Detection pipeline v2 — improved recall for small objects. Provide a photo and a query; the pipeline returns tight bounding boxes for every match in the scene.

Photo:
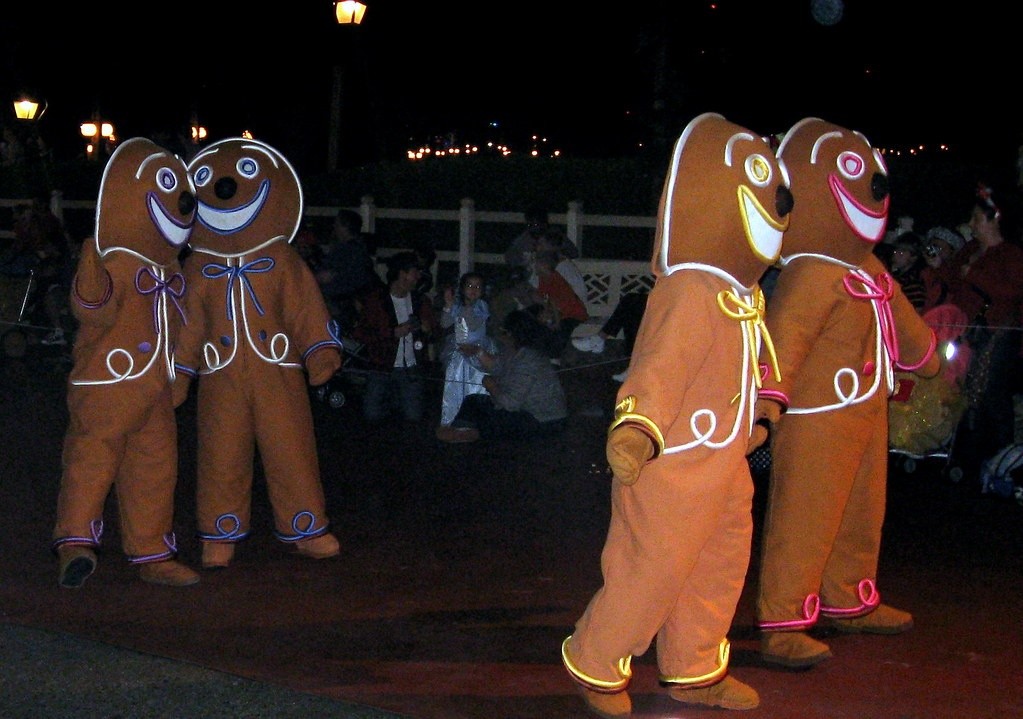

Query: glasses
[464,283,482,290]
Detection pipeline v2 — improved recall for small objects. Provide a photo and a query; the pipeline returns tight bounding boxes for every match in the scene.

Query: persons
[0,121,120,380]
[288,205,652,462]
[867,181,1023,427]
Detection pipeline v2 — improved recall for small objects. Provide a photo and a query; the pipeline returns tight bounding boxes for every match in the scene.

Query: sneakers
[571,336,604,354]
[612,369,629,382]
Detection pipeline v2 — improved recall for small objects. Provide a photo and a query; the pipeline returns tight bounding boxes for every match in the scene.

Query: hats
[888,232,920,252]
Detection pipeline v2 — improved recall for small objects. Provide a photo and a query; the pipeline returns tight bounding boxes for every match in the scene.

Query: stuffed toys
[53,137,343,588]
[562,113,939,719]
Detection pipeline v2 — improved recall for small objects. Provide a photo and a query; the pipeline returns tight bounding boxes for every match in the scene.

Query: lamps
[333,0,367,25]
[12,97,48,120]
